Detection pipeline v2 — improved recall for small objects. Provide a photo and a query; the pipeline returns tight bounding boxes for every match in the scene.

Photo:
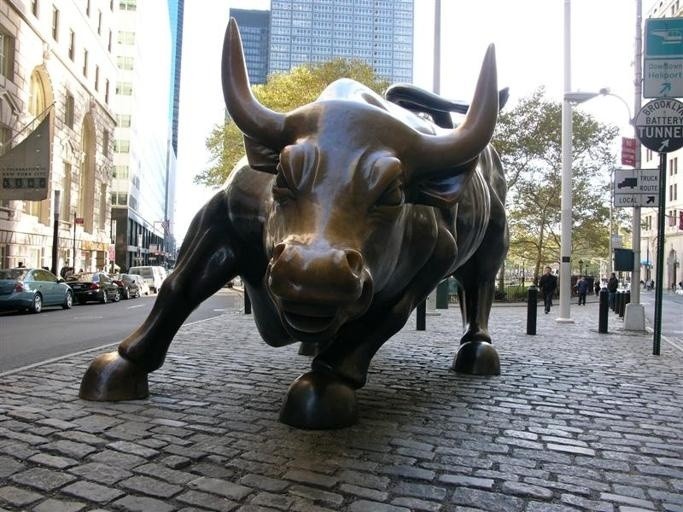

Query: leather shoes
[544,310,551,314]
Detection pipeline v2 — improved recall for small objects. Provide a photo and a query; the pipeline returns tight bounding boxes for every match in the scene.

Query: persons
[573,275,601,306]
[538,266,558,314]
[60,261,74,280]
[607,273,618,311]
[16,261,26,268]
[619,276,654,291]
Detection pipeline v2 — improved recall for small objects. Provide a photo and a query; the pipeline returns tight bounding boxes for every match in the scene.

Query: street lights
[563,87,642,303]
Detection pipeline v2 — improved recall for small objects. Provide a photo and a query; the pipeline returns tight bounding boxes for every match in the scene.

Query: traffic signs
[635,16,682,152]
[614,169,660,207]
[621,136,635,168]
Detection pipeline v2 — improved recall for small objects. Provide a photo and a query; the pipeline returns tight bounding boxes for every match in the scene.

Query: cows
[78,15,512,432]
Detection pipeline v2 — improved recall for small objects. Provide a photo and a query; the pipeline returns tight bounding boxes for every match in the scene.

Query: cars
[1,266,168,313]
